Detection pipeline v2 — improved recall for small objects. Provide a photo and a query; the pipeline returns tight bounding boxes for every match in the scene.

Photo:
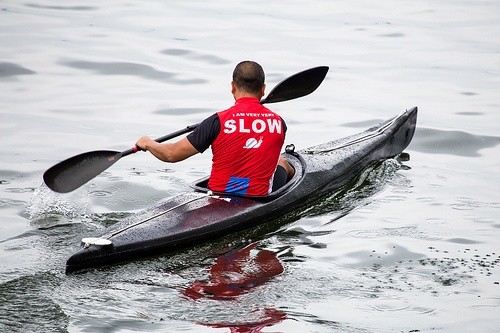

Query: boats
[64,106,418,273]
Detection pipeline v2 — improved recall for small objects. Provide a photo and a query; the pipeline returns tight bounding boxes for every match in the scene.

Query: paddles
[42,65,330,194]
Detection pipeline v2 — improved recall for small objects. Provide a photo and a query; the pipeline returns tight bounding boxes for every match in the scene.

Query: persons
[135,61,295,197]
